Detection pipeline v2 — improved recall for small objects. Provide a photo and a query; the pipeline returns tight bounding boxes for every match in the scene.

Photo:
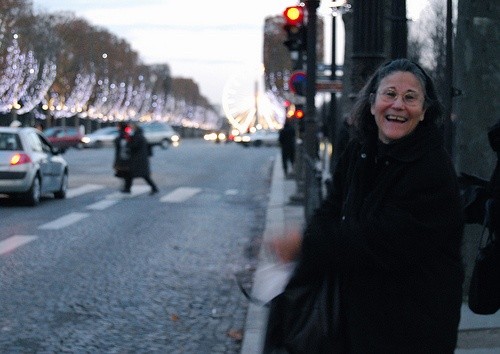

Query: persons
[278,121,296,174]
[262,57,467,354]
[112,121,160,196]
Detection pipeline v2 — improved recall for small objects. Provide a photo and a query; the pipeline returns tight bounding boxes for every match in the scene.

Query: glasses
[373,86,425,105]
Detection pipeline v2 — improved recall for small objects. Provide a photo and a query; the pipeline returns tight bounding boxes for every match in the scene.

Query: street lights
[326,0,342,140]
[7,98,21,121]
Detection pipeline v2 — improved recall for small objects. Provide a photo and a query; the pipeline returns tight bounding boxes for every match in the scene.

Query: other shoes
[118,186,130,192]
[149,185,158,195]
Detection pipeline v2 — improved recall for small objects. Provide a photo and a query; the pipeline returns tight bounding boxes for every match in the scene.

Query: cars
[139,123,180,148]
[238,131,280,146]
[0,127,69,206]
[83,126,120,148]
[42,127,84,150]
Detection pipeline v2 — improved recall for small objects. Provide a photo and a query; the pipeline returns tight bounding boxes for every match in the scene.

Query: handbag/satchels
[468,209,500,314]
[266,276,352,354]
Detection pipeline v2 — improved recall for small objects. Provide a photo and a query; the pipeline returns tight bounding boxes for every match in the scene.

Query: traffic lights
[294,105,304,138]
[283,7,307,51]
